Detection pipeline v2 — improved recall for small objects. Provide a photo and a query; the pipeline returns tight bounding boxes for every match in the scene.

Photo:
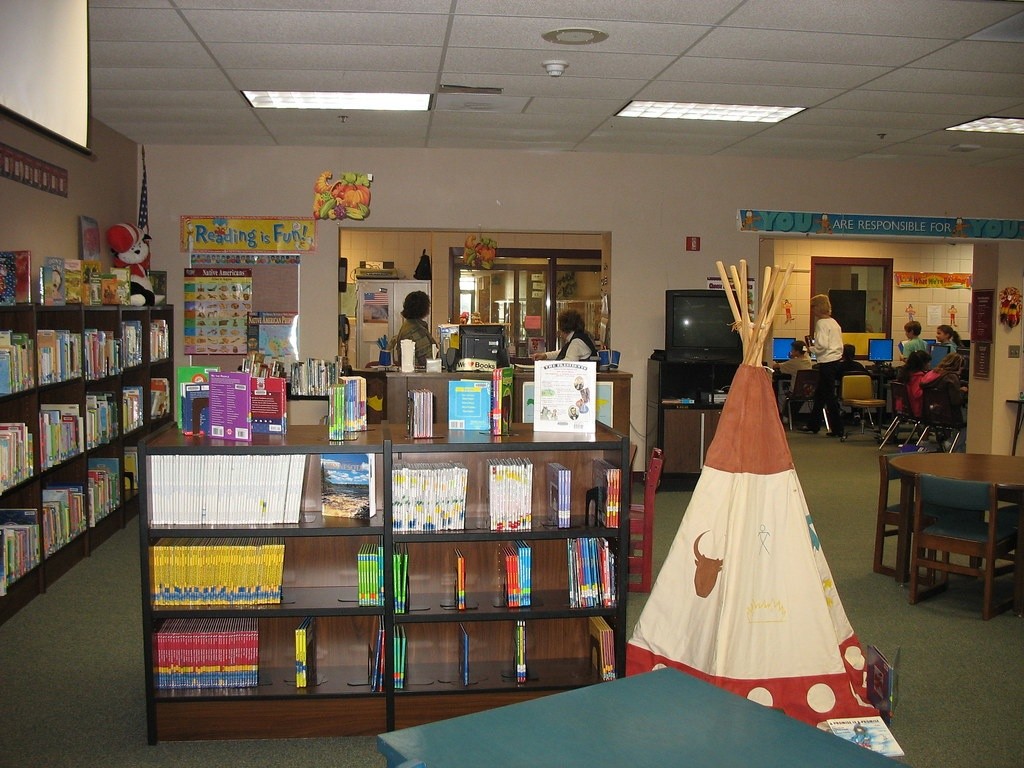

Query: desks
[1006,400,1024,456]
[376,667,907,768]
[889,453,1024,617]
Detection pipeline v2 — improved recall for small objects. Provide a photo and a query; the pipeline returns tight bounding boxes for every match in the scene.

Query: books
[43,447,138,560]
[176,366,287,442]
[392,456,621,530]
[448,367,514,435]
[241,351,352,396]
[407,388,434,438]
[328,376,366,441]
[157,618,258,689]
[357,537,617,694]
[148,454,307,525]
[38,320,169,386]
[39,378,170,472]
[817,643,905,757]
[0,508,40,596]
[153,538,285,607]
[321,453,377,519]
[0,330,34,394]
[0,423,34,496]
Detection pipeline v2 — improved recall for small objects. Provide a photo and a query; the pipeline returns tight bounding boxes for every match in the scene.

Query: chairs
[630,444,666,593]
[781,370,967,453]
[873,451,1019,620]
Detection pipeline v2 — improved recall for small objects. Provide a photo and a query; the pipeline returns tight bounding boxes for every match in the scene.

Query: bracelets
[903,358,907,362]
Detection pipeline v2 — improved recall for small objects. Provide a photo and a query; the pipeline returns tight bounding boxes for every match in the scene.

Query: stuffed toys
[106,223,165,306]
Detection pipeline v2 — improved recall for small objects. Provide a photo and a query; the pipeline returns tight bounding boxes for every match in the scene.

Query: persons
[532,309,597,361]
[773,341,812,424]
[900,321,968,453]
[796,294,845,437]
[396,290,439,366]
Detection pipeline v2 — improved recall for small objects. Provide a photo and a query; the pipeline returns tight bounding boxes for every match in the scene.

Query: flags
[138,165,150,269]
[364,293,388,305]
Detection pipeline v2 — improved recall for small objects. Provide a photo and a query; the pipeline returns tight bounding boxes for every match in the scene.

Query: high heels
[796,425,820,434]
[826,431,844,437]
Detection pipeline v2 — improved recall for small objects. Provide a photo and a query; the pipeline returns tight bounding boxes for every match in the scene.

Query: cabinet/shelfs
[645,359,767,491]
[0,304,175,627]
[356,280,431,368]
[138,419,632,744]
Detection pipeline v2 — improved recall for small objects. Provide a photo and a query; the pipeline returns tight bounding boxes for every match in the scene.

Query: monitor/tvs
[923,339,969,371]
[459,325,510,369]
[665,289,744,362]
[868,339,894,362]
[772,338,796,363]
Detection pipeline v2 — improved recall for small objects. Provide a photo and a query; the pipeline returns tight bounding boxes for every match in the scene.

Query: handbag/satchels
[413,249,431,280]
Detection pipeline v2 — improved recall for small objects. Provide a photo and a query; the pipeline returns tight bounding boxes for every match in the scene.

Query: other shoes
[941,441,952,449]
[919,440,930,447]
[851,412,860,425]
[839,410,848,425]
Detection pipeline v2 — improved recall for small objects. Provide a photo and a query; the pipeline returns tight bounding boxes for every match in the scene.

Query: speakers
[446,348,459,373]
